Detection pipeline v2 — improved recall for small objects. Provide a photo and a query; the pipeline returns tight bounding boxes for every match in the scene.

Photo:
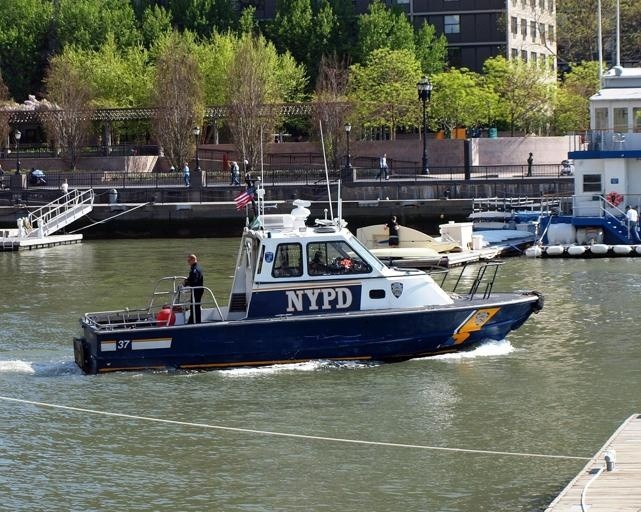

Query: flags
[234,185,257,212]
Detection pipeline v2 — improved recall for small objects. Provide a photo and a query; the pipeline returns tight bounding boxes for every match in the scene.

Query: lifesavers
[606,191,621,208]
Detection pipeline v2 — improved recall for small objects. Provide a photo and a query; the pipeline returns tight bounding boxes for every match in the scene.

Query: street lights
[344,122,352,167]
[192,125,201,171]
[15,130,22,175]
[417,75,433,175]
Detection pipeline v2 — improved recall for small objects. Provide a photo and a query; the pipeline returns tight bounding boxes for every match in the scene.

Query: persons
[375,152,391,180]
[181,253,205,325]
[245,160,252,172]
[62,179,71,205]
[182,162,192,186]
[624,205,641,243]
[383,216,401,246]
[527,152,534,177]
[232,162,241,185]
[312,251,326,269]
[0,164,6,190]
[223,151,231,171]
[229,161,238,185]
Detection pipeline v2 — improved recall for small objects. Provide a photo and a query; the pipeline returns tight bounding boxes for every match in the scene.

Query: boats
[72,121,544,375]
